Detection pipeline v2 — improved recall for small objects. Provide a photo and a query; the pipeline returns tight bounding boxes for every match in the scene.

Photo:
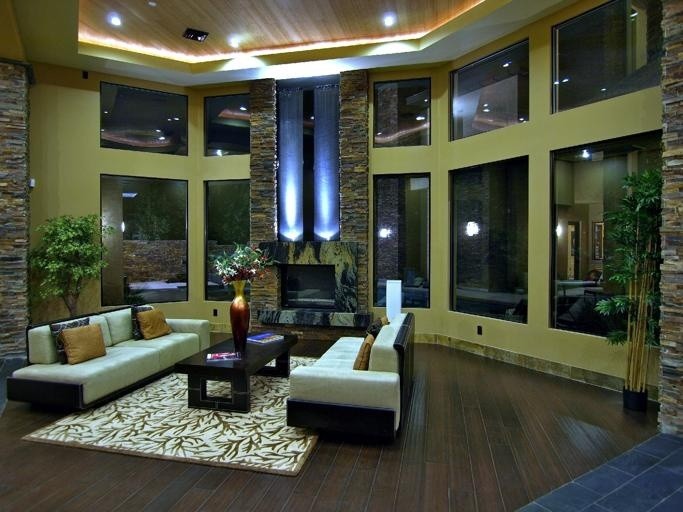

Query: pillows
[381,316,388,324]
[50,316,90,365]
[366,318,382,336]
[61,322,106,365]
[137,308,172,340]
[131,306,156,340]
[353,334,374,370]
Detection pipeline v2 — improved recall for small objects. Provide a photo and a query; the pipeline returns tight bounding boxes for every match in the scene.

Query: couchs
[6,304,212,413]
[286,312,417,448]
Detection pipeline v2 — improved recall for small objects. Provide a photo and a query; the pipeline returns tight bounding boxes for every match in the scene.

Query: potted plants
[594,171,663,416]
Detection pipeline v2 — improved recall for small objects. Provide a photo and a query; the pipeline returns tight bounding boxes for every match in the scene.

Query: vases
[226,275,256,352]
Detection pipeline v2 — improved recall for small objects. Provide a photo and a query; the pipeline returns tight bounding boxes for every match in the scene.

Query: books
[205,352,241,364]
[246,332,284,345]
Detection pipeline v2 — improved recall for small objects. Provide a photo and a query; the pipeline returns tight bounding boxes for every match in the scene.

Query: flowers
[212,243,277,284]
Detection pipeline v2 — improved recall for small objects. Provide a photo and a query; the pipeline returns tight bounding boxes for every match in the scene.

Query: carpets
[21,355,322,477]
